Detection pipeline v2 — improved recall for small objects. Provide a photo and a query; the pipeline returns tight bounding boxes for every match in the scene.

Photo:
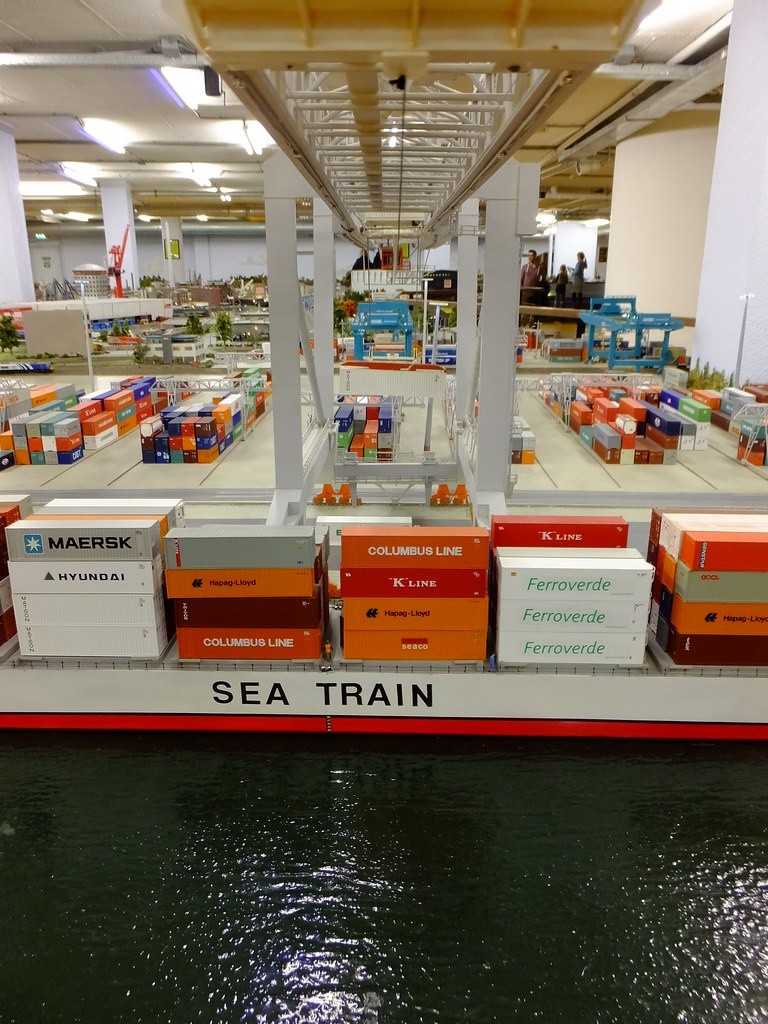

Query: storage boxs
[1,323,768,669]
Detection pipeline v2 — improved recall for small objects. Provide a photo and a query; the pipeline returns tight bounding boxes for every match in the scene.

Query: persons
[520,248,545,305]
[552,265,569,308]
[569,252,587,309]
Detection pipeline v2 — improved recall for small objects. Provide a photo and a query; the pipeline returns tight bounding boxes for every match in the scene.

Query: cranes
[105,223,130,298]
